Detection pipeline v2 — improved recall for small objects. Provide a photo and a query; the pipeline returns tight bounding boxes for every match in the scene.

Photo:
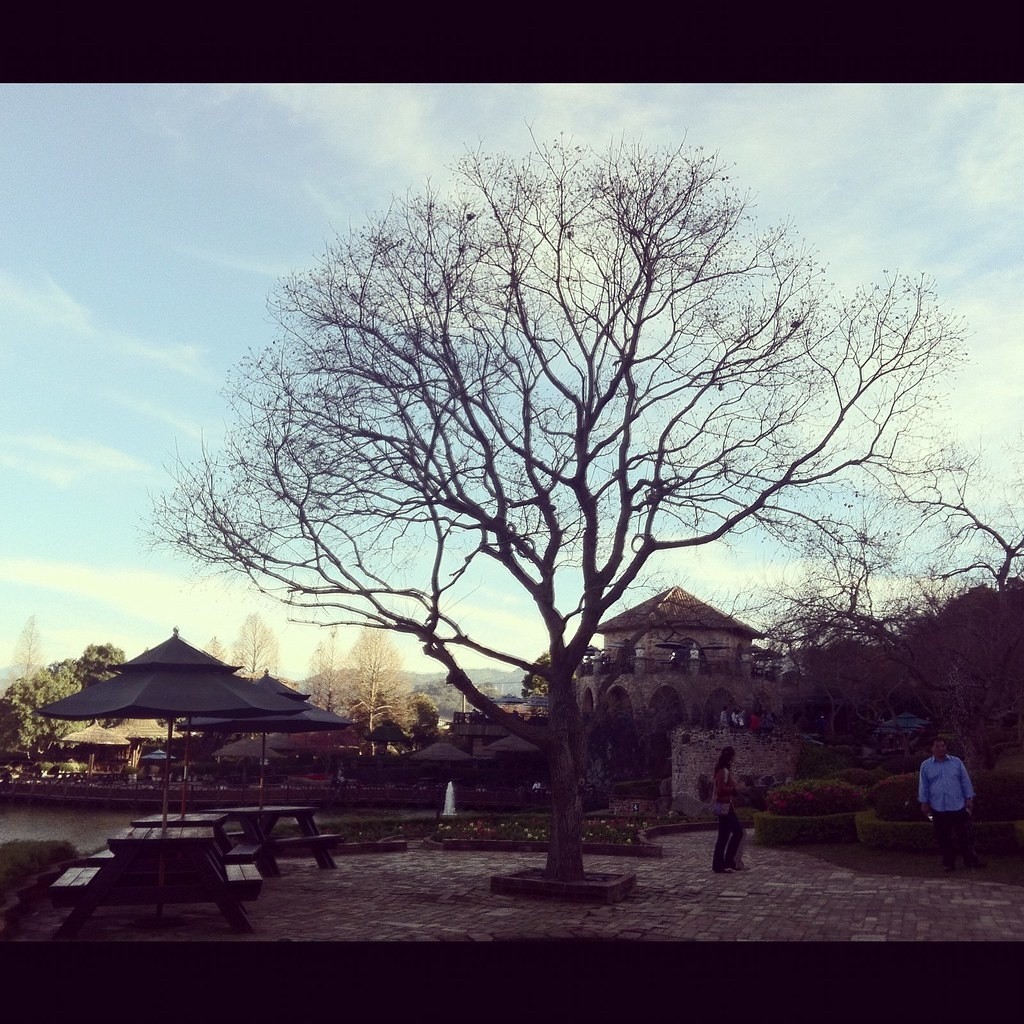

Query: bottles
[928,812,933,821]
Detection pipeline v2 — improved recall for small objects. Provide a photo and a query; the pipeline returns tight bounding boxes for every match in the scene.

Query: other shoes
[722,868,736,873]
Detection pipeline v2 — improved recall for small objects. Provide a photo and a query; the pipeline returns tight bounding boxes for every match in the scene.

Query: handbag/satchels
[713,803,734,817]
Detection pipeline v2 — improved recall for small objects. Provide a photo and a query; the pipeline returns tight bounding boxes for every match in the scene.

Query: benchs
[50,866,101,907]
[227,831,244,840]
[256,833,344,875]
[223,841,264,860]
[88,848,115,865]
[226,863,263,893]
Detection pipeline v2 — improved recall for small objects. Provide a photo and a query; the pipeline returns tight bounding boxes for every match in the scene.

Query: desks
[52,826,253,936]
[129,813,233,856]
[199,805,339,876]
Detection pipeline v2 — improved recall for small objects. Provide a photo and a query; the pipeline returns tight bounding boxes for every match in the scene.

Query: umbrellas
[36,627,311,830]
[177,668,353,809]
[61,717,536,792]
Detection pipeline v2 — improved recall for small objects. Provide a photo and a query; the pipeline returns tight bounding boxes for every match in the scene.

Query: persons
[720,705,776,737]
[711,746,746,873]
[919,736,979,873]
